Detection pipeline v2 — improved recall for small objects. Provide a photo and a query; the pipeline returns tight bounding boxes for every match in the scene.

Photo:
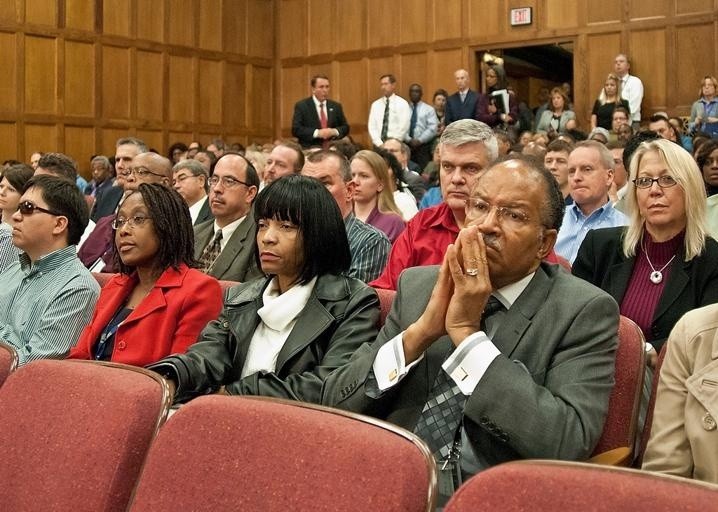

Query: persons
[1,108,718,347]
[68,184,222,371]
[292,64,520,151]
[642,303,717,486]
[688,74,718,137]
[589,53,644,131]
[570,139,717,465]
[317,154,619,512]
[509,83,576,137]
[1,173,101,391]
[146,173,382,413]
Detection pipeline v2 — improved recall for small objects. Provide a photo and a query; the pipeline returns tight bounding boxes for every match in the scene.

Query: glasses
[207,176,250,186]
[464,198,530,227]
[631,175,675,188]
[120,167,165,177]
[16,201,60,216]
[110,216,153,229]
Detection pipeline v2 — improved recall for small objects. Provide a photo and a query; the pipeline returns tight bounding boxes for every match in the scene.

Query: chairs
[0,340,18,382]
[128,395,439,511]
[440,458,718,512]
[87,274,669,463]
[1,357,173,511]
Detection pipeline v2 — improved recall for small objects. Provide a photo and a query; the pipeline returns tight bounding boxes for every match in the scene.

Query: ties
[380,100,388,143]
[197,229,222,275]
[409,104,416,137]
[319,103,329,150]
[413,296,501,463]
